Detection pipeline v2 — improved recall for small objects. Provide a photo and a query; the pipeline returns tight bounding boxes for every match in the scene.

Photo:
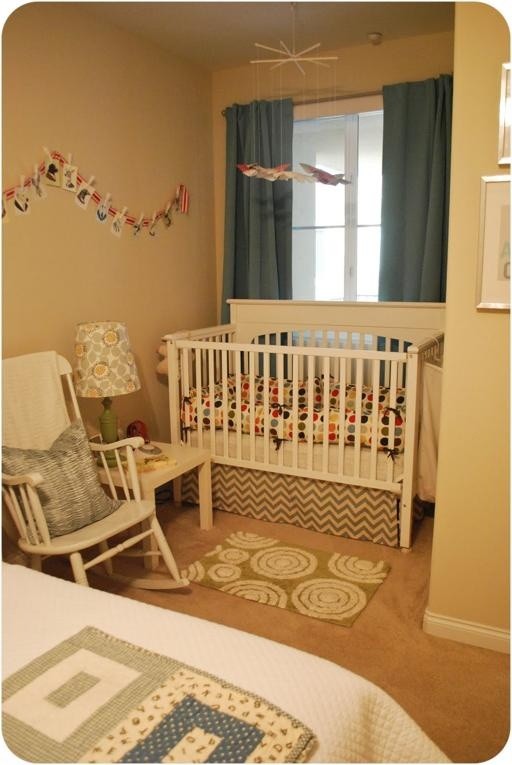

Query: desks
[88,436,214,571]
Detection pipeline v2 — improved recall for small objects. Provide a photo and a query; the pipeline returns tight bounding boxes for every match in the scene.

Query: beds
[4,561,383,762]
[161,296,446,546]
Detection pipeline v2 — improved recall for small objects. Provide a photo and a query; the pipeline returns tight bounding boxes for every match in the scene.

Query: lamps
[71,319,145,470]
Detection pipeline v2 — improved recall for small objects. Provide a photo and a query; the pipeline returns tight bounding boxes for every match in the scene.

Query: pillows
[1,418,124,545]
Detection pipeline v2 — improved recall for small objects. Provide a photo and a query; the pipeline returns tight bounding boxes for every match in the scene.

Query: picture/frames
[475,62,511,312]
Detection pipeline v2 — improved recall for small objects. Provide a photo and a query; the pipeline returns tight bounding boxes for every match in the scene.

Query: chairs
[1,350,192,593]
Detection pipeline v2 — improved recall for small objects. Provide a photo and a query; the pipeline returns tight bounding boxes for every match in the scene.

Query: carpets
[176,530,391,629]
